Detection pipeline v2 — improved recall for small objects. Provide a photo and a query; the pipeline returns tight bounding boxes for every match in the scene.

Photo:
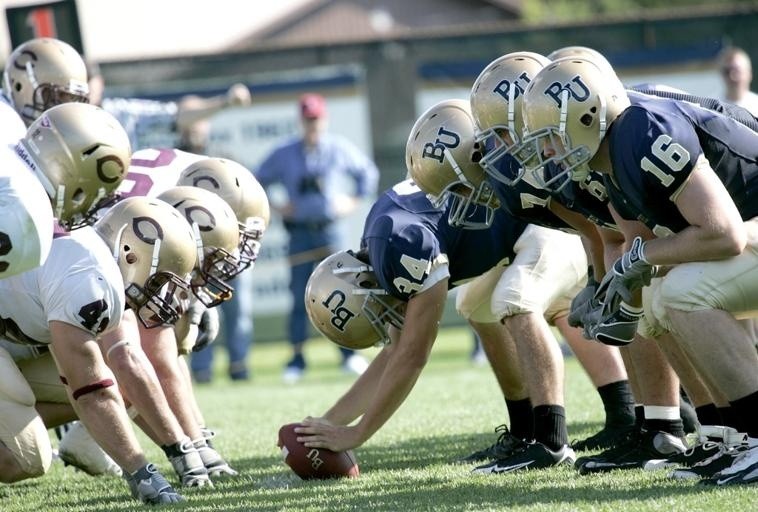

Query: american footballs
[278,423,360,481]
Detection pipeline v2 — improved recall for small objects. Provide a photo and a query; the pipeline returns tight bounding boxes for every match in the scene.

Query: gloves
[122,462,186,505]
[191,438,241,478]
[160,437,215,488]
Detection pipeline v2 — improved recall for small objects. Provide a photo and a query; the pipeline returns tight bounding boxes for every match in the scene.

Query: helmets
[304,250,406,351]
[404,98,502,231]
[12,101,132,232]
[469,51,552,187]
[156,186,242,308]
[544,45,608,62]
[520,55,632,194]
[173,157,271,283]
[92,195,198,330]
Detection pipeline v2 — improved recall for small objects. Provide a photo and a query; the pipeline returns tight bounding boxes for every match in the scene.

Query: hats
[298,92,326,119]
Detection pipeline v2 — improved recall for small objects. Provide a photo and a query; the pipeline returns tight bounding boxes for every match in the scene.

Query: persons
[0,102,131,277]
[291,174,575,474]
[471,50,758,475]
[258,95,380,377]
[0,196,217,503]
[0,187,242,488]
[713,46,758,120]
[176,92,253,383]
[55,147,271,475]
[405,98,645,474]
[515,58,758,485]
[549,46,691,97]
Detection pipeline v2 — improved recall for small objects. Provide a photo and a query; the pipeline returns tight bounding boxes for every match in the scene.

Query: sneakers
[569,428,629,453]
[573,431,639,468]
[193,368,210,383]
[579,417,686,476]
[340,354,369,375]
[281,361,304,382]
[699,445,758,487]
[229,361,247,380]
[643,424,738,472]
[458,423,536,465]
[668,428,757,480]
[469,441,576,476]
